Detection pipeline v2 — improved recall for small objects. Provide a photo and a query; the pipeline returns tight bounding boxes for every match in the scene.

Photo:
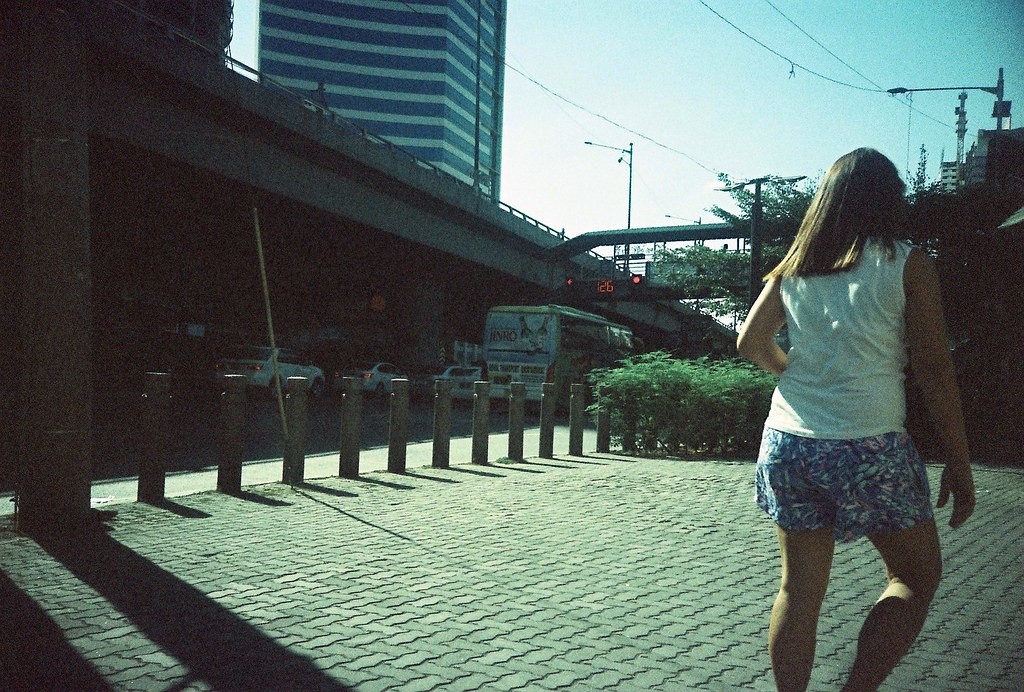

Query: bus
[481,303,642,415]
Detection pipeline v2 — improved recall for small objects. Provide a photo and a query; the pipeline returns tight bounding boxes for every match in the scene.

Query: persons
[737,148,977,692]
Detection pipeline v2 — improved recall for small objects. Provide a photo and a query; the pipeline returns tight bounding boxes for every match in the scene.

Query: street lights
[886,66,1006,188]
[584,139,634,270]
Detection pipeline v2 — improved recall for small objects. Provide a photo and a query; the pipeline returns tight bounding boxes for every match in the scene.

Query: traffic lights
[567,279,574,284]
[370,294,387,310]
[632,275,640,284]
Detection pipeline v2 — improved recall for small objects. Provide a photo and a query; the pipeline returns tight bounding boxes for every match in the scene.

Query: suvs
[206,342,327,399]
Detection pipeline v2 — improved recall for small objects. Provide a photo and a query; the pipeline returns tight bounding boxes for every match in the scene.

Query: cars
[443,365,488,400]
[333,359,407,398]
